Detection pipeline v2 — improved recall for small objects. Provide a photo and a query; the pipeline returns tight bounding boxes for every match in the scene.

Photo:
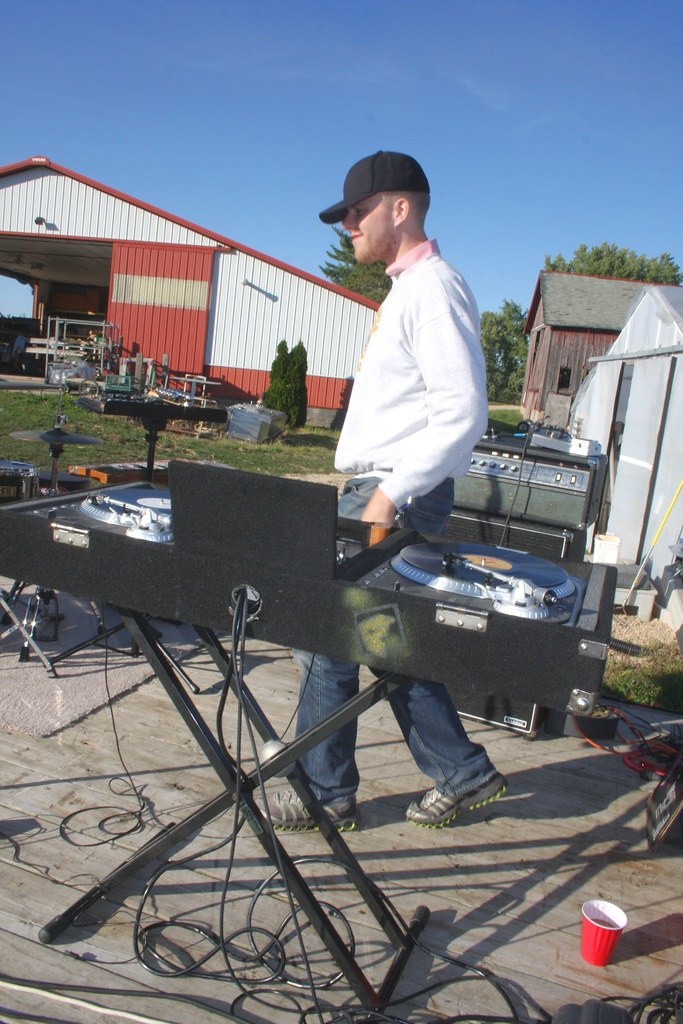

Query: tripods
[0,418,200,694]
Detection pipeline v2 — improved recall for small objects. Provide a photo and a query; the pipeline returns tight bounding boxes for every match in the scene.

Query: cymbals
[4,427,107,445]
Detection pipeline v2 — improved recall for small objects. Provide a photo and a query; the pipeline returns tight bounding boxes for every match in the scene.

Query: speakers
[433,508,588,740]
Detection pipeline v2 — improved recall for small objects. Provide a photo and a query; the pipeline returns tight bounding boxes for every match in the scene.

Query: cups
[582,899,628,965]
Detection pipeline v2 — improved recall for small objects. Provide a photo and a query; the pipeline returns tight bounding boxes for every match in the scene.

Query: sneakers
[403,771,508,828]
[253,788,360,833]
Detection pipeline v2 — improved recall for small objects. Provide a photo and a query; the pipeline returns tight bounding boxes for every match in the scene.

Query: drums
[0,458,40,504]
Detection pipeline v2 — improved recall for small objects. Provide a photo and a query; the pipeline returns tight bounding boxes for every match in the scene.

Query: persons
[255,149,507,832]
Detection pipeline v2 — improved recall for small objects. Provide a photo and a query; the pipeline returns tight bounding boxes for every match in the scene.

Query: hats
[318,150,431,224]
